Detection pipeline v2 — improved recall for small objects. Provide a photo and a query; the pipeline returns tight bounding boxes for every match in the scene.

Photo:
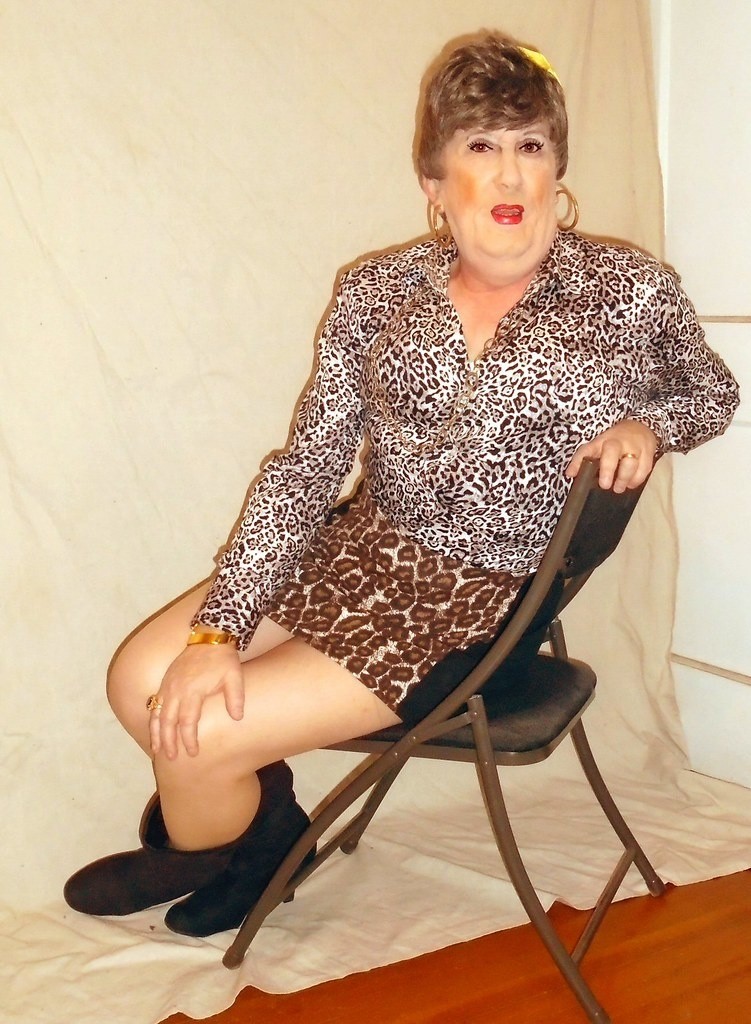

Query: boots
[164,759,318,937]
[63,774,265,917]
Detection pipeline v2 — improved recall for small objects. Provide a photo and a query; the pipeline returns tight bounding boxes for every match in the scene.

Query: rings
[621,453,635,459]
[146,695,163,711]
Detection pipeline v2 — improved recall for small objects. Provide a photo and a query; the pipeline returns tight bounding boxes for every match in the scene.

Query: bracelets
[186,632,237,646]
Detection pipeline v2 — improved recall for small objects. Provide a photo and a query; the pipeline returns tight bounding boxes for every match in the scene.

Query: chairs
[222,453,669,1024]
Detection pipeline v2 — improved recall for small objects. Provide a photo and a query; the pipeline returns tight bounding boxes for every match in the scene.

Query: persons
[64,32,742,938]
